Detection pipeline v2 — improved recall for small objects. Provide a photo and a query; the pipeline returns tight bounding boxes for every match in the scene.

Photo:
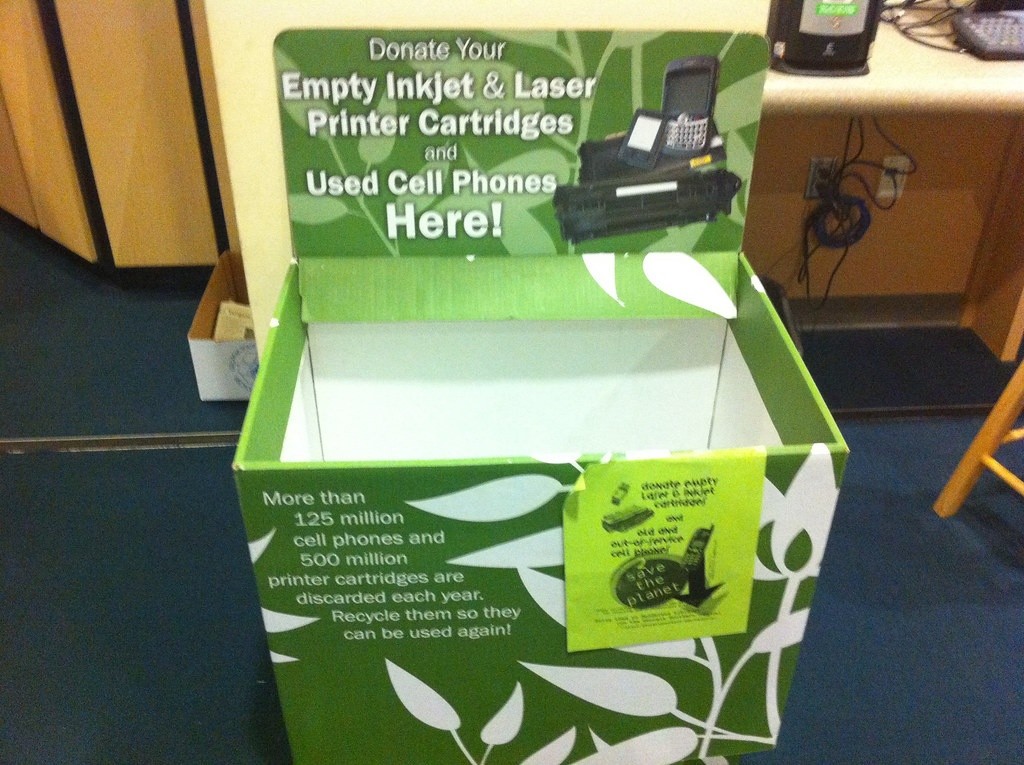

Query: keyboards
[953,9,1024,61]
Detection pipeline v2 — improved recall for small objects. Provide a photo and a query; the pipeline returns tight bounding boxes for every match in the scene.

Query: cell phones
[662,55,720,156]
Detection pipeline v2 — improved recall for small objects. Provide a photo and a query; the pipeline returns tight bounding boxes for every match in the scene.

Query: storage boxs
[232,28,851,764]
[185,248,260,400]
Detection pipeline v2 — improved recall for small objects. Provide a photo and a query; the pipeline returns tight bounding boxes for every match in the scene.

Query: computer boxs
[765,0,883,76]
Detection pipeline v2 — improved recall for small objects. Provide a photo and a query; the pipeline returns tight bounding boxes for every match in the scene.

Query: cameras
[617,107,667,170]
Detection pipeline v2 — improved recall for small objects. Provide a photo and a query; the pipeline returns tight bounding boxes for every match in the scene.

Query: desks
[206,2,1024,362]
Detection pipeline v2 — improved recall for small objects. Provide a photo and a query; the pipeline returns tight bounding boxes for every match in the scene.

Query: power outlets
[804,154,841,200]
[875,153,912,200]
